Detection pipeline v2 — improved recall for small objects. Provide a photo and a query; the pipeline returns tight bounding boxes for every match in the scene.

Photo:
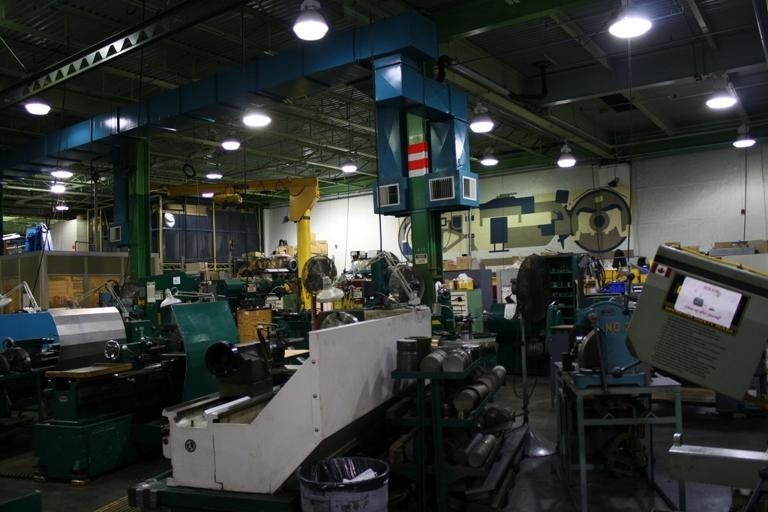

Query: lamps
[293,0,329,42]
[607,0,652,38]
[704,78,737,109]
[469,103,494,133]
[556,144,576,169]
[480,145,499,166]
[341,154,357,173]
[205,98,273,179]
[23,95,53,120]
[732,128,756,148]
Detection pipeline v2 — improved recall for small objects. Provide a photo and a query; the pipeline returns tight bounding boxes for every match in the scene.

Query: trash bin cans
[296,457,391,512]
